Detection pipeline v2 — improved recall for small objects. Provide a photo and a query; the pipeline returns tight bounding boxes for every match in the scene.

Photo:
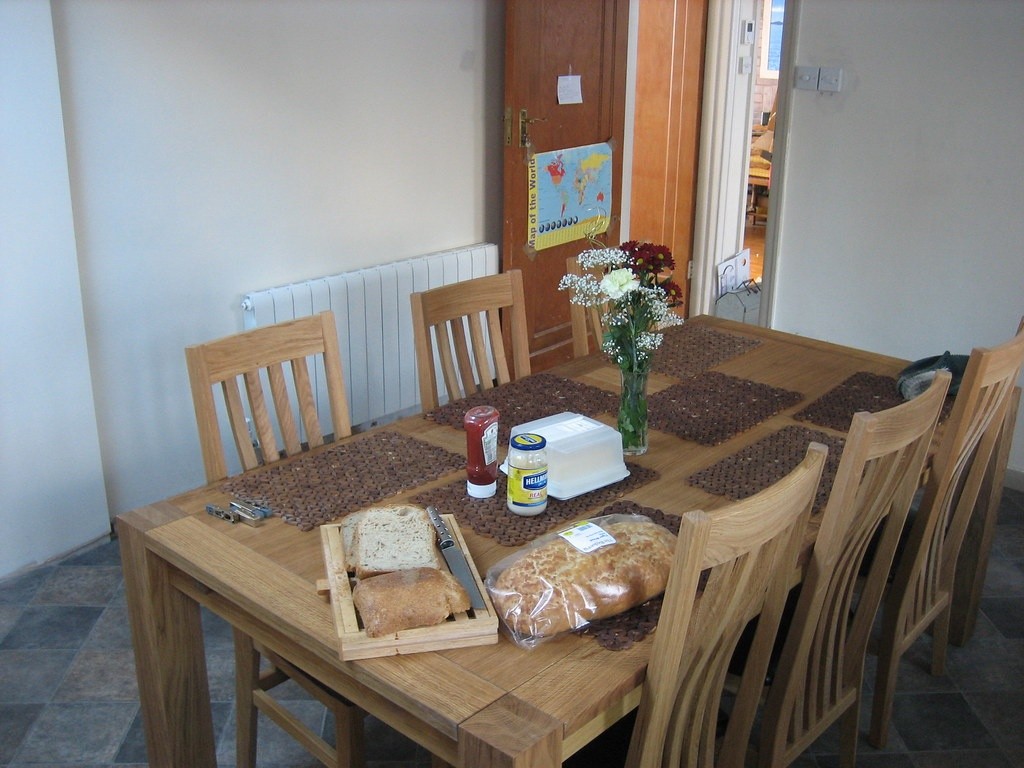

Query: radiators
[241,241,500,455]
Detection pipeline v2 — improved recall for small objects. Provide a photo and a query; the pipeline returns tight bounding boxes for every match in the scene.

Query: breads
[490,520,677,636]
[338,503,470,638]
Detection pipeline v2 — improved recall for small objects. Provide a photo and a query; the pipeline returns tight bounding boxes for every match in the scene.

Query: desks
[126,314,1022,768]
[748,176,769,226]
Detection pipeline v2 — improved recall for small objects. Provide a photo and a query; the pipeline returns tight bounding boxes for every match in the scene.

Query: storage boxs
[499,411,631,501]
[748,193,769,224]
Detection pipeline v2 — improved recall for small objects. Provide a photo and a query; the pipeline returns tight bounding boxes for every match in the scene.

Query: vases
[618,369,649,456]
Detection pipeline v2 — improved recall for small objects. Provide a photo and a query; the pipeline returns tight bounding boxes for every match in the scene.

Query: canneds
[506,433,548,516]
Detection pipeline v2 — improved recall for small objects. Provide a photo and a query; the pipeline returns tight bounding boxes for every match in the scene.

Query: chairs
[408,268,532,413]
[716,370,951,768]
[185,309,366,768]
[624,440,830,768]
[565,256,655,358]
[847,312,1024,751]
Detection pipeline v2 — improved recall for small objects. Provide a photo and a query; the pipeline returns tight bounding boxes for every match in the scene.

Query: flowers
[558,240,684,449]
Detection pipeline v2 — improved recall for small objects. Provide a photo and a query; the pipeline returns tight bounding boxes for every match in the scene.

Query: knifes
[426,505,487,613]
